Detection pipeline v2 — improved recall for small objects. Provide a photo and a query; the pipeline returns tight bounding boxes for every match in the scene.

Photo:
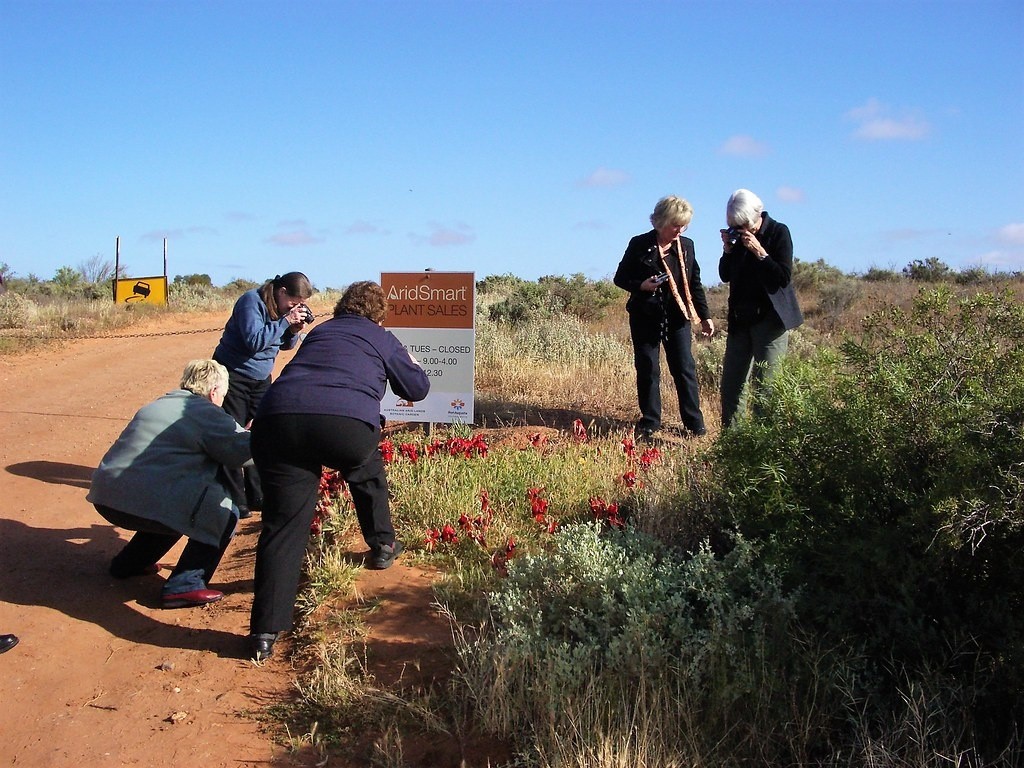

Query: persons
[212,272,313,518]
[614,197,713,436]
[251,281,432,661]
[85,360,253,609]
[714,189,802,445]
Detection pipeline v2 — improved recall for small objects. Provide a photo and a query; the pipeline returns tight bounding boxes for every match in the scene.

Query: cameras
[724,225,744,249]
[297,305,314,325]
[653,272,670,284]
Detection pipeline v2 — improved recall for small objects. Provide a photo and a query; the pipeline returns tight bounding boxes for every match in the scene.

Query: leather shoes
[164,590,223,609]
[0,634,20,653]
[110,564,162,577]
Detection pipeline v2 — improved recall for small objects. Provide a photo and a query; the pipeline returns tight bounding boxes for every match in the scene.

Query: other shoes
[634,425,660,438]
[238,505,251,518]
[242,633,273,659]
[373,541,406,569]
[695,427,707,435]
[248,497,265,510]
